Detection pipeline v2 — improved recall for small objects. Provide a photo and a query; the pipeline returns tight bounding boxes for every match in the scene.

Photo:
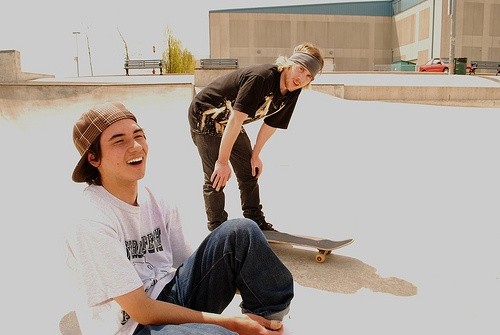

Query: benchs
[124,60,164,76]
[468,61,500,76]
[200,59,239,70]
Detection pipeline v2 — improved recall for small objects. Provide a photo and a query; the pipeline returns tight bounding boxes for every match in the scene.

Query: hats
[70,102,138,183]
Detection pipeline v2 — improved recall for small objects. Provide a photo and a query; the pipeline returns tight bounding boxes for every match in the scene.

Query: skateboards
[261,229,355,264]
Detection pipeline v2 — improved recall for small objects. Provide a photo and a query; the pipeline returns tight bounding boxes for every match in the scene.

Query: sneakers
[255,217,280,232]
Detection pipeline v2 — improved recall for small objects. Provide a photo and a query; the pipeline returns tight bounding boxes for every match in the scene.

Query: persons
[56,103,296,335]
[188,43,324,233]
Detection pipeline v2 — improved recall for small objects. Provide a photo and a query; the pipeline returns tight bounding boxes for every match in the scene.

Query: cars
[419,57,471,74]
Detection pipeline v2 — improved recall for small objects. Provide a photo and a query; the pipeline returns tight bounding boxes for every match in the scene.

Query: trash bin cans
[453,57,467,74]
